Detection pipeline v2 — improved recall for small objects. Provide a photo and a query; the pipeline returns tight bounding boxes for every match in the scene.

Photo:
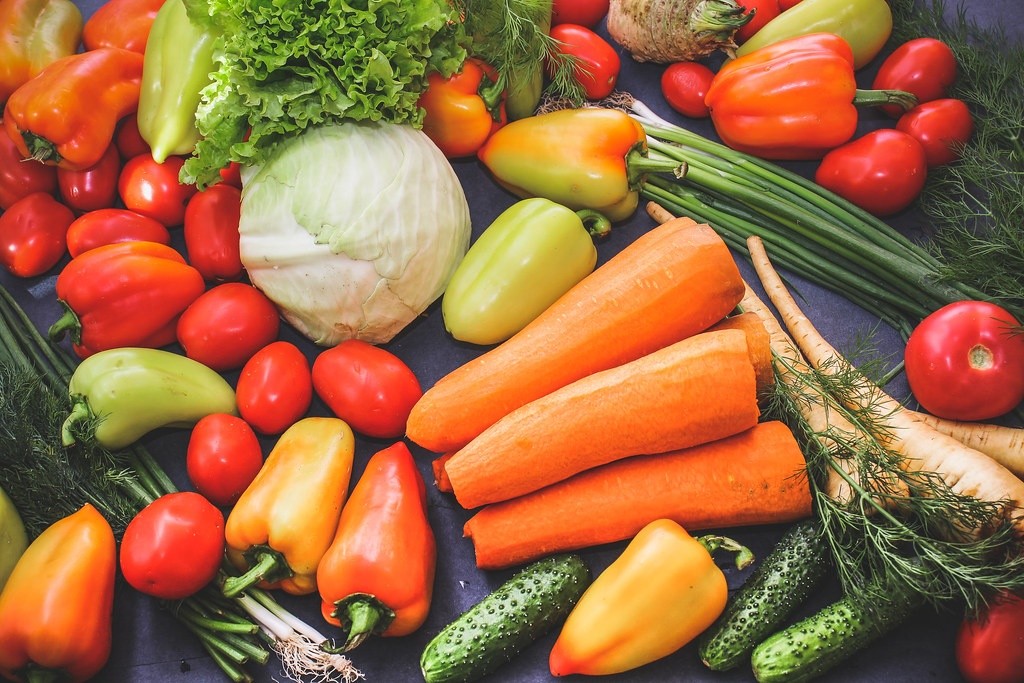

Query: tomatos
[952,593,1024,683]
[545,0,975,216]
[0,106,421,602]
[904,301,1024,420]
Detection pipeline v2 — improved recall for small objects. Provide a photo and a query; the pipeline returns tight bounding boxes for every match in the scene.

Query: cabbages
[240,119,473,346]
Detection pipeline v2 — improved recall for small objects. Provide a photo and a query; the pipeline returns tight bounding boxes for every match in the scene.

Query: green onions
[0,284,366,683]
[538,92,1023,387]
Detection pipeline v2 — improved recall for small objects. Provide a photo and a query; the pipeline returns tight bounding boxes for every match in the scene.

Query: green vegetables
[715,298,1023,632]
[882,1,1023,342]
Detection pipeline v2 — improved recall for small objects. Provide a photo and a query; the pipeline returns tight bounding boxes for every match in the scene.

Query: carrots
[639,196,1023,539]
[403,218,815,566]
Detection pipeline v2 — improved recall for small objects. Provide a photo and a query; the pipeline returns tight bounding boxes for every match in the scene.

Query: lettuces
[177,0,468,191]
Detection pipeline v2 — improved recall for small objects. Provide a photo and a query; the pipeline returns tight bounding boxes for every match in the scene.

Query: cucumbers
[421,519,932,683]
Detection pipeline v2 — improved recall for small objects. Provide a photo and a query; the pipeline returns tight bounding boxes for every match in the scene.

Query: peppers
[418,58,509,156]
[477,108,691,224]
[316,439,436,654]
[550,520,755,677]
[718,0,897,75]
[219,417,355,598]
[0,0,239,450]
[704,32,921,163]
[500,0,552,120]
[443,197,612,346]
[0,502,117,683]
